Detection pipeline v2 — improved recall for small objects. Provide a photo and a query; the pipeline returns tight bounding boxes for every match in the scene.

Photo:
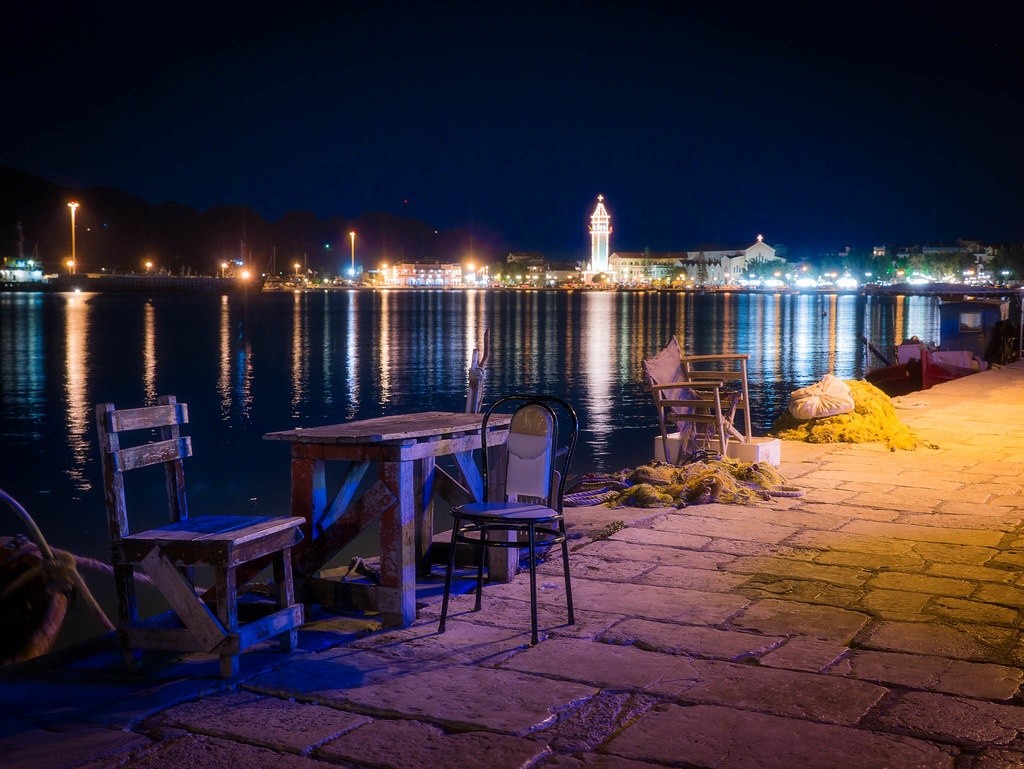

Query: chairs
[642,335,752,463]
[436,394,581,643]
[96,394,306,685]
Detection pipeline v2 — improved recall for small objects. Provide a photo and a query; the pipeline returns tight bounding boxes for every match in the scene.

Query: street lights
[349,231,357,279]
[68,199,80,272]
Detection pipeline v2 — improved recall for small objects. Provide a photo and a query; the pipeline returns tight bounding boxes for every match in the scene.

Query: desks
[262,411,517,627]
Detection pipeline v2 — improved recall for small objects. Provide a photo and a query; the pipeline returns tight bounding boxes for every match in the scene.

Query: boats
[0,490,75,672]
[857,299,1020,397]
